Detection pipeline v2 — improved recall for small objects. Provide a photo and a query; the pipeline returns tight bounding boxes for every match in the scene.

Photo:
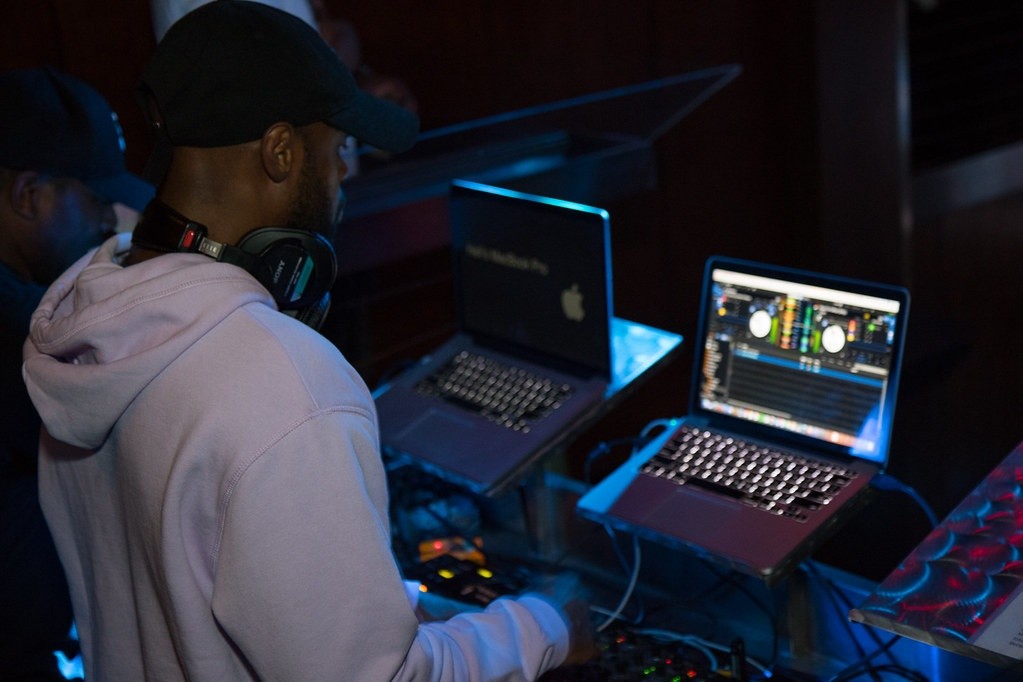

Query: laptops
[576,254,910,580]
[373,178,617,490]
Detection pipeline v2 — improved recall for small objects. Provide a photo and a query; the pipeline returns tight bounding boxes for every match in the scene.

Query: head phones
[130,197,339,333]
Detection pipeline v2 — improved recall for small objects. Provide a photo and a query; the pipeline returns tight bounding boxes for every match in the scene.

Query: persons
[21,0,596,682]
[0,48,155,682]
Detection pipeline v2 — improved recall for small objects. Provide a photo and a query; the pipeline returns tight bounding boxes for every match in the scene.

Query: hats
[140,0,420,152]
[0,71,166,210]
